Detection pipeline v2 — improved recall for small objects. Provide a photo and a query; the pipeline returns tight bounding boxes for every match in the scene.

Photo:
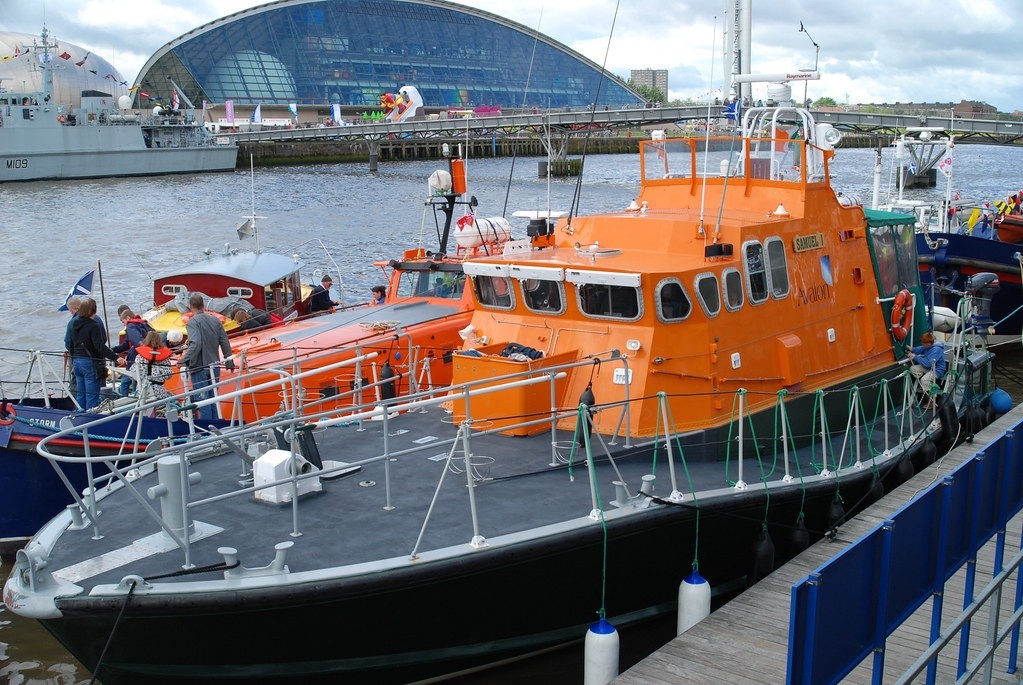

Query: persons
[231,307,272,331]
[176,295,235,420]
[379,93,406,114]
[371,285,387,305]
[111,305,183,418]
[310,274,343,319]
[905,333,946,409]
[64,297,128,414]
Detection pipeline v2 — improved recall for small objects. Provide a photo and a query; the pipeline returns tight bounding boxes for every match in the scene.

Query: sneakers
[923,401,933,409]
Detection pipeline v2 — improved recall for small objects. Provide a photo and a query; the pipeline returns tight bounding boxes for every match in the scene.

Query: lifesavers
[181,310,226,325]
[891,289,913,341]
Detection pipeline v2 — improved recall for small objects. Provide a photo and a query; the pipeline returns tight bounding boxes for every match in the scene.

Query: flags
[59,52,71,60]
[117,81,131,87]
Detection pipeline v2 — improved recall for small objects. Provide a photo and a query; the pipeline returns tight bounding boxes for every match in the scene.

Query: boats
[106,246,324,371]
[877,190,1023,363]
[2,101,998,685]
[0,151,589,564]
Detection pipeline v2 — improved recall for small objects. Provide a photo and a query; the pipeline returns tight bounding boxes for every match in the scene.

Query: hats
[321,275,333,282]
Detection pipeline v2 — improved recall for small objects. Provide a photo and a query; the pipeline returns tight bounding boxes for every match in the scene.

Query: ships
[0,22,241,183]
